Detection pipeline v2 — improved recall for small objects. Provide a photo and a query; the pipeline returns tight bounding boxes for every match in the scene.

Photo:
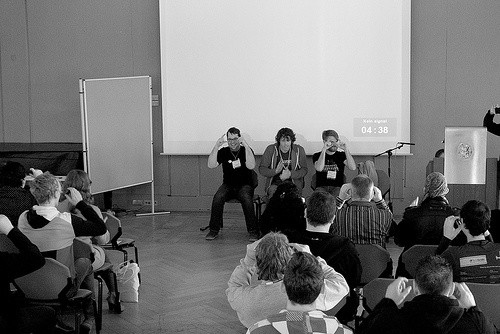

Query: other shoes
[80,321,92,334]
[205,230,217,240]
[248,231,258,241]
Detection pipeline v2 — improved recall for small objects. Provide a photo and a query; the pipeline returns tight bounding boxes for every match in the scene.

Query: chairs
[101,212,141,284]
[221,192,262,232]
[13,257,98,334]
[73,238,122,331]
[376,170,394,212]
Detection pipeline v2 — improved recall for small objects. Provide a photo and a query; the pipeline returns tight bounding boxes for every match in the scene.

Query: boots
[105,273,120,311]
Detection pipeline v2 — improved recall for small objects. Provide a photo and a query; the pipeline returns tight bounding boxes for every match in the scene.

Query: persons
[483,104,500,136]
[205,127,259,242]
[330,175,393,278]
[259,182,307,237]
[288,191,363,322]
[246,252,354,334]
[258,128,308,236]
[394,172,467,280]
[434,200,500,284]
[354,255,500,334]
[225,231,350,329]
[0,214,56,334]
[0,161,43,227]
[57,169,124,314]
[312,130,357,197]
[18,173,106,334]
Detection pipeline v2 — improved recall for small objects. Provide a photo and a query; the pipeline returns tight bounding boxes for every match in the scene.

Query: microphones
[398,142,415,146]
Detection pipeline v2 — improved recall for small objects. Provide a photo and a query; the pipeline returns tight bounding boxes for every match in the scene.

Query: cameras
[495,108,500,114]
[453,219,462,228]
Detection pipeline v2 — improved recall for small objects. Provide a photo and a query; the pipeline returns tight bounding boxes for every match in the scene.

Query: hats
[425,172,447,196]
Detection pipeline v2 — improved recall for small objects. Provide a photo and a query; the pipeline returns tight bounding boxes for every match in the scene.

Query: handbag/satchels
[117,260,140,302]
